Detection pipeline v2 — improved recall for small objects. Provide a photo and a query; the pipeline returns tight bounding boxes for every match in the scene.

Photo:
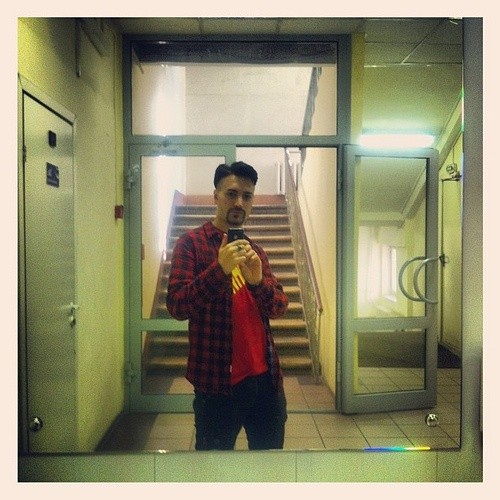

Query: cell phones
[227,228,244,243]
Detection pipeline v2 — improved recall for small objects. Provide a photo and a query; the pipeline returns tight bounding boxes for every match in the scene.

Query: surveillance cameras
[447,163,458,178]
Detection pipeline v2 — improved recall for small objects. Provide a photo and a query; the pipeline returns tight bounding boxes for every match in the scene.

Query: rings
[238,244,242,251]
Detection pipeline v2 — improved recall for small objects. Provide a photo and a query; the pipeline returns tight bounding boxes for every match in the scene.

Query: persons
[165,161,288,451]
